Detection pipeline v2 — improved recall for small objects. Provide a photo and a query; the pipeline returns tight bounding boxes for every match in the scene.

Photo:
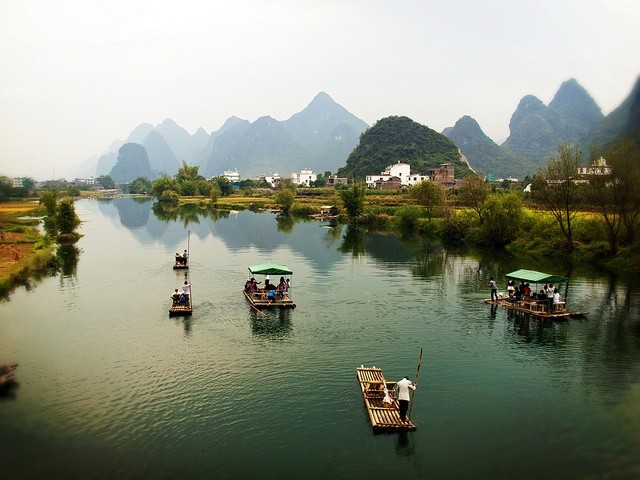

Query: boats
[485,269,587,318]
[173,232,189,270]
[170,272,192,313]
[356,364,417,431]
[0,360,20,386]
[243,263,296,307]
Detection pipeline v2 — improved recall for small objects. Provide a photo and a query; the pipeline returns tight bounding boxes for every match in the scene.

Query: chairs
[383,381,397,397]
[175,256,181,264]
[172,294,180,305]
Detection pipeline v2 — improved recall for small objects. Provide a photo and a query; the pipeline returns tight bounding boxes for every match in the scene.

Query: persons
[175,252,182,265]
[520,281,525,293]
[286,279,290,294]
[264,275,270,285]
[281,276,284,279]
[540,283,562,310]
[512,286,522,302]
[397,376,417,420]
[506,277,515,294]
[488,277,499,302]
[268,289,276,305]
[182,281,190,307]
[182,249,188,266]
[524,283,531,298]
[171,288,180,307]
[246,278,261,292]
[278,279,286,300]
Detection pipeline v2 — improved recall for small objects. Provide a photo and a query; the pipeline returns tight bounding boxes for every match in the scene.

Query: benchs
[365,381,383,395]
[252,289,289,300]
[519,300,545,312]
